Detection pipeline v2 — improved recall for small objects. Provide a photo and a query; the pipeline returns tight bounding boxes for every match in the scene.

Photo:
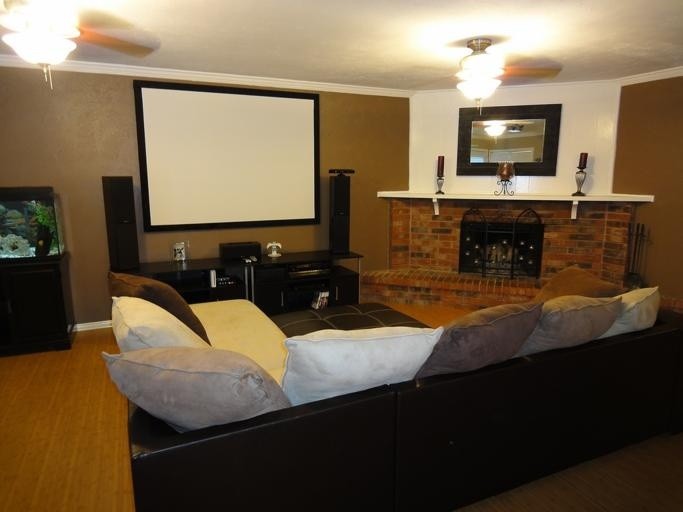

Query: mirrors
[456,104,562,176]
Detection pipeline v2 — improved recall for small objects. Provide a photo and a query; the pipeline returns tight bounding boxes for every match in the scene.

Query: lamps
[0,0,80,91]
[484,125,507,145]
[454,38,505,117]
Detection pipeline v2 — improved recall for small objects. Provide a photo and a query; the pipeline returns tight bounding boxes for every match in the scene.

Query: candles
[437,156,444,177]
[580,153,588,168]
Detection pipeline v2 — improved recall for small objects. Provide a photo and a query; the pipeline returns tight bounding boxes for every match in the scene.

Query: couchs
[126,300,682,512]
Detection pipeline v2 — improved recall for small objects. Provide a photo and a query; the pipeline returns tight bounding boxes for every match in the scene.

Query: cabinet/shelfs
[119,248,250,317]
[249,250,364,316]
[0,253,76,357]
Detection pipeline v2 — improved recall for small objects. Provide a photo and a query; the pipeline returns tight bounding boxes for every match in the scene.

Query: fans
[74,29,154,60]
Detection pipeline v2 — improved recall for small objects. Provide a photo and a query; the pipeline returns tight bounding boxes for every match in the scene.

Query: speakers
[330,176,350,255]
[102,176,140,271]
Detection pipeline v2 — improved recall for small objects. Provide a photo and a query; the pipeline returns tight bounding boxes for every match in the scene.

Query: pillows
[107,270,213,347]
[99,347,291,432]
[109,293,213,350]
[598,285,662,340]
[534,263,629,301]
[510,293,623,361]
[412,300,543,380]
[267,325,446,407]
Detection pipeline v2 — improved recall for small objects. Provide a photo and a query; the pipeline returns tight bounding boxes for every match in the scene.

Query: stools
[269,302,432,337]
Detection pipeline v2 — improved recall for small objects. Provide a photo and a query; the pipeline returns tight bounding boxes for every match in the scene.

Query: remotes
[250,256,258,262]
[241,256,251,263]
